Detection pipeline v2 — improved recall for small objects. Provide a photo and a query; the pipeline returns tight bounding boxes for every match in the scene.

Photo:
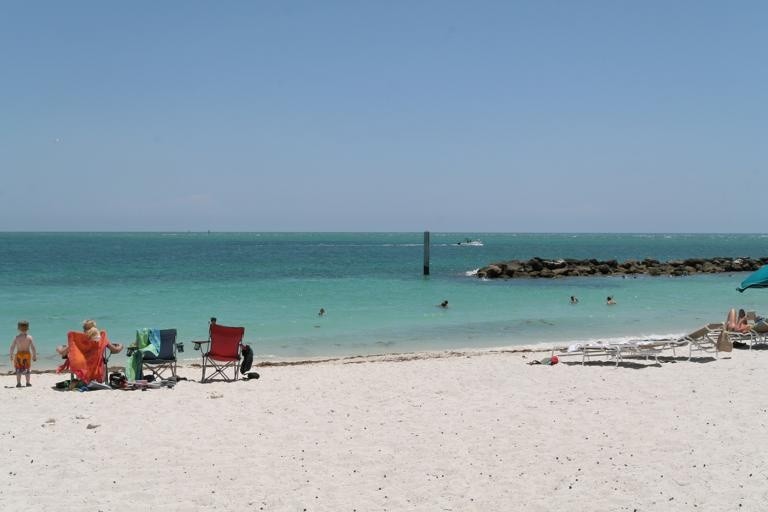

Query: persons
[8,319,38,389]
[55,317,124,362]
[605,296,616,305]
[569,296,579,305]
[726,306,765,333]
[738,308,747,326]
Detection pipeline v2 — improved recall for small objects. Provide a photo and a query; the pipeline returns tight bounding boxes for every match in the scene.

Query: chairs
[551,316,768,367]
[65,325,245,384]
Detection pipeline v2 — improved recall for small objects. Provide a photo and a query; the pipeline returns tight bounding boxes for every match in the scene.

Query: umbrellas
[735,264,768,294]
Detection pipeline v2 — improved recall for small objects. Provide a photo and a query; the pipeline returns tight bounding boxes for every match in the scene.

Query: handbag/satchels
[716,325,733,353]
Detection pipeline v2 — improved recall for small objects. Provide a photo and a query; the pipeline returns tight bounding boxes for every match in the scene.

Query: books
[745,311,757,321]
